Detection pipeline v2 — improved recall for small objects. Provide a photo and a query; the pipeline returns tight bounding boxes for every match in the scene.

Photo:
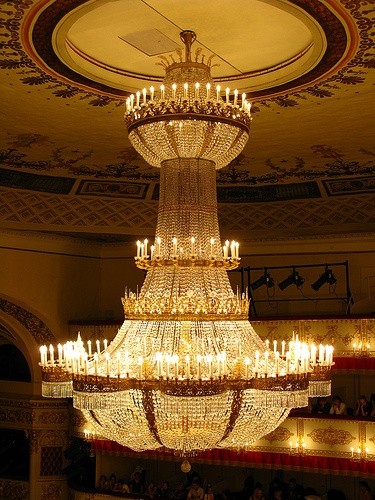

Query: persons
[97,472,234,500]
[249,487,265,500]
[358,481,374,500]
[329,395,347,415]
[353,395,372,417]
[272,487,285,500]
[240,470,254,500]
[312,397,330,414]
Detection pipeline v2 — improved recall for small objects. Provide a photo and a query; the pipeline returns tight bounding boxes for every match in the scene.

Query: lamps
[38,26,333,472]
[309,266,337,292]
[251,266,273,291]
[277,264,307,292]
